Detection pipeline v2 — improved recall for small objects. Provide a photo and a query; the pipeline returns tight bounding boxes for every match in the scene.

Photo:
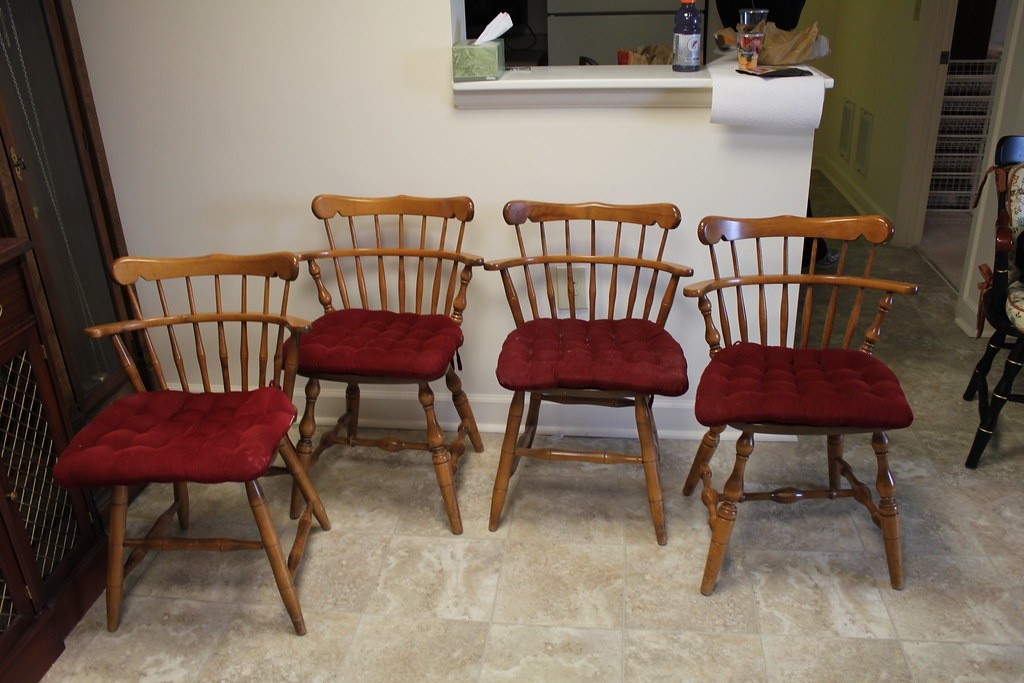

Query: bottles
[671,0,703,73]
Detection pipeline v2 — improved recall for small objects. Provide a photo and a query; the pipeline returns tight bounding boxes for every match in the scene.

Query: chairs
[680,214,914,596]
[288,194,484,536]
[482,201,694,552]
[962,133,1023,470]
[51,251,330,636]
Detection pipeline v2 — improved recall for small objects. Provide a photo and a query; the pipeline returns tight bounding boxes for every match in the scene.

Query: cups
[736,33,764,69]
[738,9,768,33]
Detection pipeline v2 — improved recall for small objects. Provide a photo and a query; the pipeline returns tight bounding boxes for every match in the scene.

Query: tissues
[452,12,514,83]
